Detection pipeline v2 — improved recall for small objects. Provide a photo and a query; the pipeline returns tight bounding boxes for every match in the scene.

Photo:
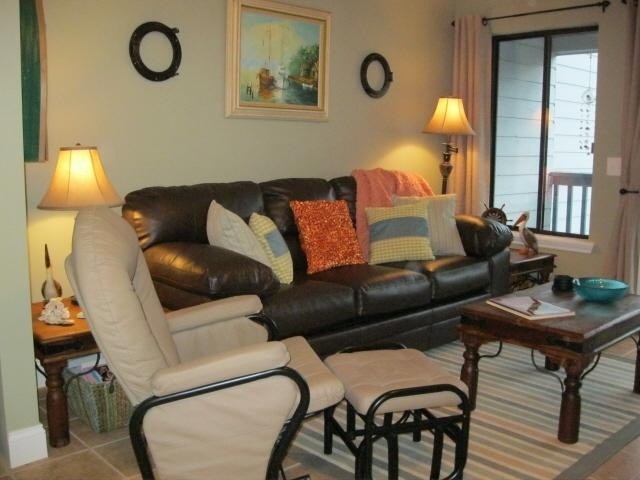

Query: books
[486,294,576,322]
[65,352,115,385]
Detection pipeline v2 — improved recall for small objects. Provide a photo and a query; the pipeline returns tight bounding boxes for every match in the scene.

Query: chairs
[63,199,347,479]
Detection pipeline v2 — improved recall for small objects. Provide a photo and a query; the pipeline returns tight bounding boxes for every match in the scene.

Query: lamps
[423,98,478,194]
[38,145,124,211]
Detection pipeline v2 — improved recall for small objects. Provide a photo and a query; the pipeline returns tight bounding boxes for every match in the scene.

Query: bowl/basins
[572,276,629,303]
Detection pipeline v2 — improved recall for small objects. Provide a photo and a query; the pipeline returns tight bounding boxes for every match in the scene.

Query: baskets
[63,365,136,433]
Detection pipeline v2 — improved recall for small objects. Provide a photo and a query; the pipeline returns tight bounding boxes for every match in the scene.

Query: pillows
[204,195,467,286]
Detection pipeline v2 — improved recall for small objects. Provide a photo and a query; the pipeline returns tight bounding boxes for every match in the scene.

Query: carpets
[287,338,636,478]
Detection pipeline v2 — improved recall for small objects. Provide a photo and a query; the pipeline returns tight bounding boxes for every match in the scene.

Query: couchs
[124,173,514,353]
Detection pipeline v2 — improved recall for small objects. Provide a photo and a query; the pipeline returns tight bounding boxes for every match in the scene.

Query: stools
[323,339,470,479]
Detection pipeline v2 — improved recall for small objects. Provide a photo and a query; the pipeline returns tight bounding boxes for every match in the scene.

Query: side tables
[32,295,105,446]
[509,251,557,288]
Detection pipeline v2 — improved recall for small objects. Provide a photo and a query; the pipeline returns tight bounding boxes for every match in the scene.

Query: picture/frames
[225,0,333,124]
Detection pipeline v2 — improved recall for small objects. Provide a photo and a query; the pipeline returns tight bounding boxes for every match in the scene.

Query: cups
[552,274,573,292]
